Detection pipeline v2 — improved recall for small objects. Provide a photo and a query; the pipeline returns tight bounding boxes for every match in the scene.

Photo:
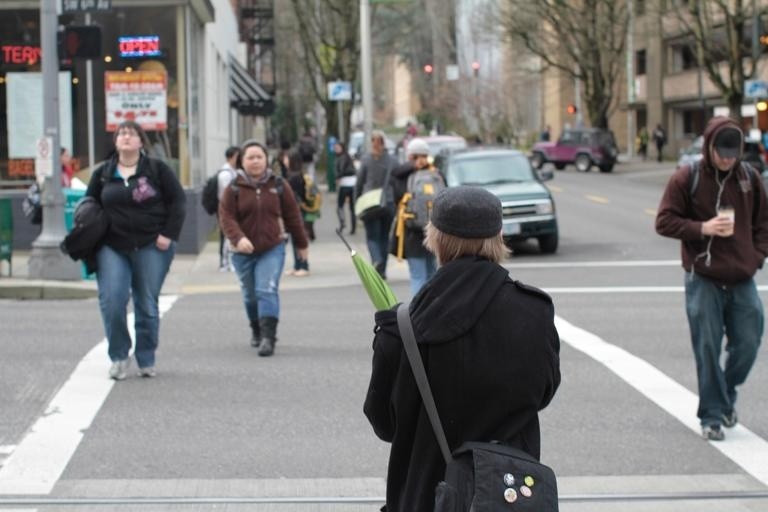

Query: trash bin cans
[0,198,13,273]
[62,187,99,280]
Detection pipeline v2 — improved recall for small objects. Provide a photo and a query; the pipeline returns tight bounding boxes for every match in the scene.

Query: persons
[406,122,415,137]
[219,139,309,357]
[360,184,562,512]
[353,131,401,280]
[391,137,449,299]
[60,145,73,188]
[654,115,767,441]
[538,114,670,165]
[75,122,186,379]
[334,141,357,234]
[217,134,322,276]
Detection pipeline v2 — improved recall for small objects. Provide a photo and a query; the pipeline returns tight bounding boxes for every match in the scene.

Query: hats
[407,137,431,155]
[429,183,504,239]
[714,126,741,159]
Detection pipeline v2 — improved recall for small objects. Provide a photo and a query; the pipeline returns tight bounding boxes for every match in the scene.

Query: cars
[675,127,766,177]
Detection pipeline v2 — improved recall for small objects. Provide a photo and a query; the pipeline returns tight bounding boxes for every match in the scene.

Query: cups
[717,206,734,238]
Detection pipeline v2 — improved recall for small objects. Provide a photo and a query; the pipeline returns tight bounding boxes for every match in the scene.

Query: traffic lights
[66,27,101,59]
[471,60,479,76]
[567,104,577,115]
[424,59,433,79]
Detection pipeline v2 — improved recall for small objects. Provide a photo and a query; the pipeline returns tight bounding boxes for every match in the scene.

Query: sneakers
[719,406,739,427]
[108,355,132,381]
[701,420,726,442]
[284,266,313,277]
[136,364,156,378]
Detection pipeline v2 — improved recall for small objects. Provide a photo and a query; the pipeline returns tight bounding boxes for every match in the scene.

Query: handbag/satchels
[352,186,388,218]
[432,440,559,512]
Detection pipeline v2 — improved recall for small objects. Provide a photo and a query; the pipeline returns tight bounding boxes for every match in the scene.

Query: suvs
[345,130,558,252]
[527,127,619,174]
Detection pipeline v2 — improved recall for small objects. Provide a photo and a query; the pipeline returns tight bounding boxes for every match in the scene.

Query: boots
[258,315,280,356]
[244,315,263,348]
